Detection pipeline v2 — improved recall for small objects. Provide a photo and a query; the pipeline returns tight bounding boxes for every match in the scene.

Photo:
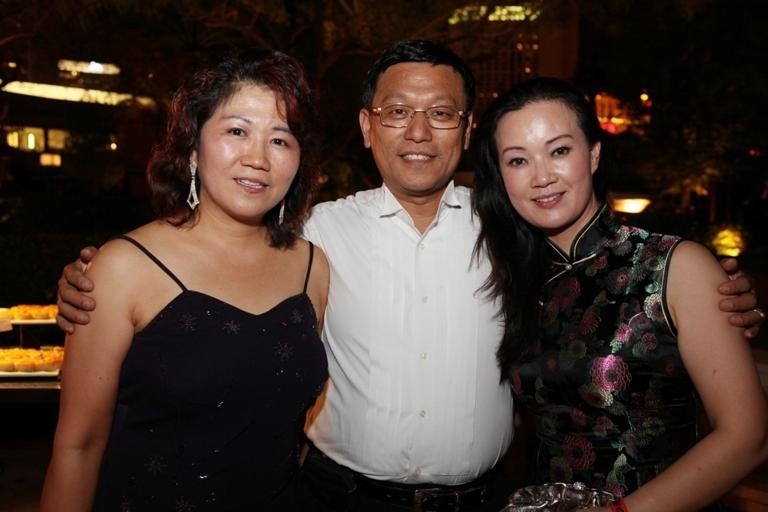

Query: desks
[0,380,60,512]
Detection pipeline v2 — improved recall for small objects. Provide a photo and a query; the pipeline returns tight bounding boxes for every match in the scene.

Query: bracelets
[607,495,628,512]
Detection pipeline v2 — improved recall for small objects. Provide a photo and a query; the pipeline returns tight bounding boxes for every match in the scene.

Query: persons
[51,40,766,511]
[466,74,767,510]
[35,51,331,512]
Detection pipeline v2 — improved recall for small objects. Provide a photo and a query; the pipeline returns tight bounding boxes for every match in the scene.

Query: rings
[752,310,764,320]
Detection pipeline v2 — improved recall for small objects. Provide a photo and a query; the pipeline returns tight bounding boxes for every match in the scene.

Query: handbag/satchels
[498,482,618,511]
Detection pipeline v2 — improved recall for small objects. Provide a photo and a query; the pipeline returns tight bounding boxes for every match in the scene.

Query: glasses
[368,103,468,130]
